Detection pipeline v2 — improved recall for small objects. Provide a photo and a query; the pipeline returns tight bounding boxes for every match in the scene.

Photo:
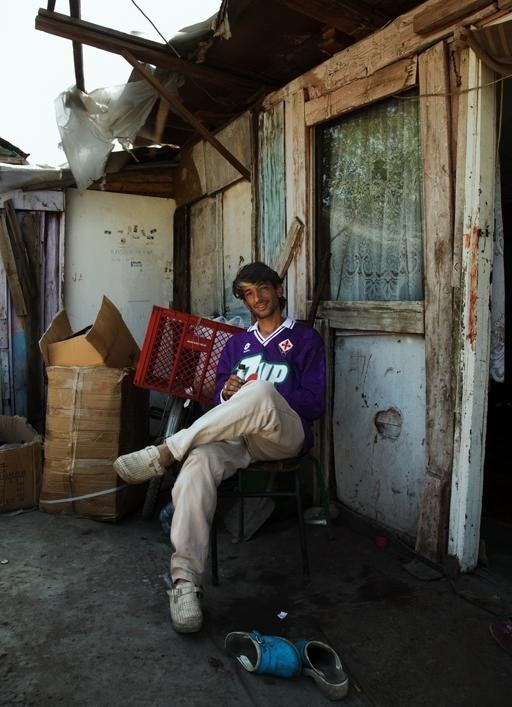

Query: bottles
[236,364,246,383]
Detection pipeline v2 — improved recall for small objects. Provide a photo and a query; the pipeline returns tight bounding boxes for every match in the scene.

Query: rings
[224,384,227,389]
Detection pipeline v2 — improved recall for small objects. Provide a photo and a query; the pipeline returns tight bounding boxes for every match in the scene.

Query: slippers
[293,638,348,701]
[223,630,302,677]
[164,582,204,633]
[114,444,168,485]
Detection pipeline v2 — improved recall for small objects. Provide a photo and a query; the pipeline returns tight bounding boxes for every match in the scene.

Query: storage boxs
[39,366,150,521]
[0,414,42,513]
[38,295,142,368]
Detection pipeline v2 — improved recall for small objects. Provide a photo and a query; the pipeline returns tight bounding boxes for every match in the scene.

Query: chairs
[210,451,331,586]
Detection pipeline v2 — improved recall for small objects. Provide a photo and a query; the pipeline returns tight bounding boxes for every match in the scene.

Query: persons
[112,262,327,637]
